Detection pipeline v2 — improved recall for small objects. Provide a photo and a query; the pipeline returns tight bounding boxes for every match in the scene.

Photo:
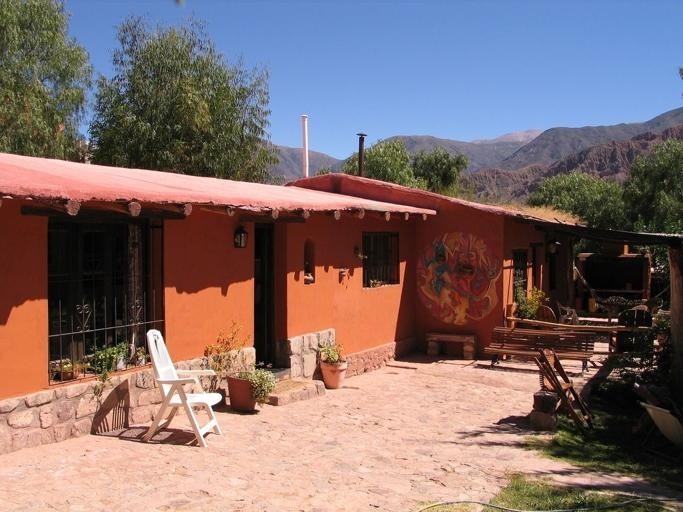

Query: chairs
[139,328,221,448]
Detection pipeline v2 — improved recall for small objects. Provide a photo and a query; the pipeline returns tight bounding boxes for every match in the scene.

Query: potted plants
[316,340,347,389]
[225,361,278,414]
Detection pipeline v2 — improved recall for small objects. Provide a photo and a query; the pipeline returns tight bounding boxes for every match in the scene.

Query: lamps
[232,224,250,248]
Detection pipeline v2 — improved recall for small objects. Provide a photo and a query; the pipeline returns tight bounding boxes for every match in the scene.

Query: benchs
[482,326,595,376]
